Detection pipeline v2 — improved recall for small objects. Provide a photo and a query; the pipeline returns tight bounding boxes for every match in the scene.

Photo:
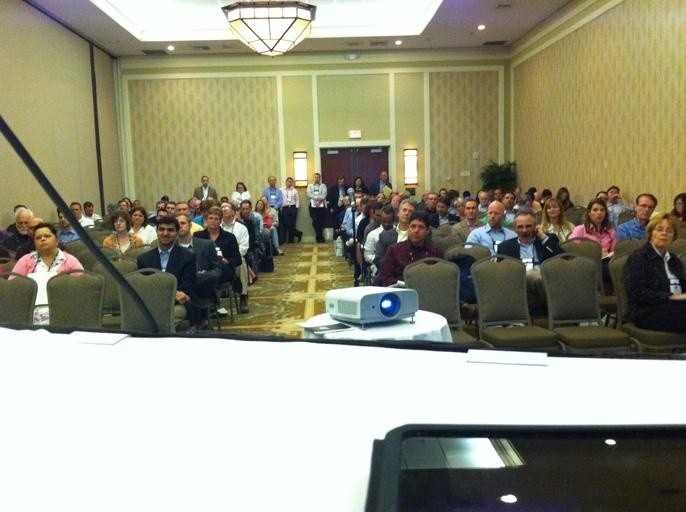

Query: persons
[352,176,370,194]
[137,194,284,330]
[231,182,251,208]
[102,197,158,255]
[334,185,686,334]
[369,170,392,196]
[306,173,327,243]
[57,200,103,243]
[279,177,302,243]
[262,176,283,245]
[8,222,85,325]
[6,204,66,261]
[329,175,350,244]
[194,176,217,200]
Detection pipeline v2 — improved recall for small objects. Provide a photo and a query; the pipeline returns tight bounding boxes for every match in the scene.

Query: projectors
[325,285,419,328]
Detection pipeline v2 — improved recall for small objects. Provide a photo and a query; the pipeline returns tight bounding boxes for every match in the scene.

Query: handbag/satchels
[333,228,349,241]
[334,235,344,256]
[260,256,274,272]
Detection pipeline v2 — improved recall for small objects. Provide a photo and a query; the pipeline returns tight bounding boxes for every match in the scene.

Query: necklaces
[31,251,59,273]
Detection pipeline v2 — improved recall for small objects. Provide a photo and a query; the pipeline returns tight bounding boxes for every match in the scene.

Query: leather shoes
[274,248,284,256]
[297,231,303,241]
[252,272,257,282]
[249,278,254,286]
[239,295,249,313]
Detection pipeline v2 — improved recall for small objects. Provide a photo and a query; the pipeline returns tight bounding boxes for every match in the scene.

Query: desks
[301,309,454,344]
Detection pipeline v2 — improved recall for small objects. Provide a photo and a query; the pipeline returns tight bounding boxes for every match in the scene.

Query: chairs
[401,205,686,325]
[0,214,273,334]
[471,254,558,353]
[609,252,685,354]
[540,252,632,354]
[402,257,493,352]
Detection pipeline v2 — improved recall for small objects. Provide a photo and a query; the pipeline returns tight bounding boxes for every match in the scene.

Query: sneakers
[211,306,227,319]
[345,237,358,247]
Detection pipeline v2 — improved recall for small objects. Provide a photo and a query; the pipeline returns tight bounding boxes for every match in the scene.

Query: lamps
[220,1,317,59]
[293,151,309,189]
[403,148,419,196]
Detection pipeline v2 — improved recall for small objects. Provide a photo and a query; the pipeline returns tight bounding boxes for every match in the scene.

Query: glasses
[221,206,232,211]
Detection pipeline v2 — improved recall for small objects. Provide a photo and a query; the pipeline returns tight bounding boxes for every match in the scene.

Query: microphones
[347,188,356,211]
